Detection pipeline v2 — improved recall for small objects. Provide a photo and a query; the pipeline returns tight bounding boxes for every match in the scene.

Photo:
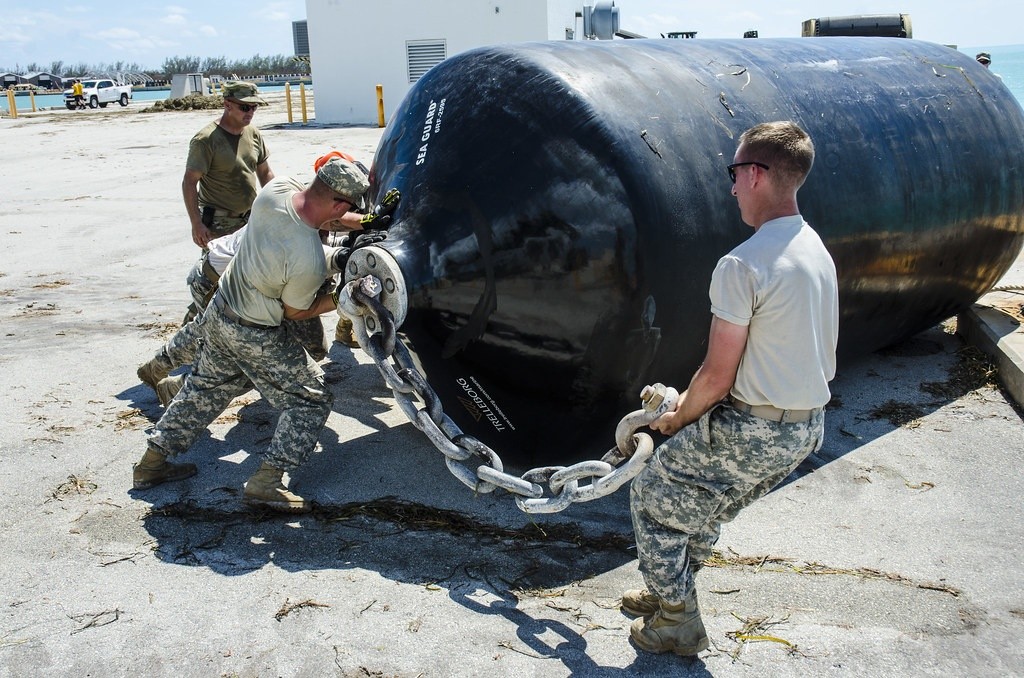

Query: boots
[132,449,197,490]
[336,319,361,348]
[156,373,185,409]
[242,462,309,513]
[631,588,710,656]
[622,588,700,616]
[137,359,168,390]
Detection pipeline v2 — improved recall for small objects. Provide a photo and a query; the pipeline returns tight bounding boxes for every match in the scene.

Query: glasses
[333,196,358,212]
[980,61,988,63]
[727,162,769,183]
[227,100,258,112]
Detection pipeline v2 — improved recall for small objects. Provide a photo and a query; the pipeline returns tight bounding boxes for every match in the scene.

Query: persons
[182,79,275,323]
[620,120,841,654]
[976,52,991,67]
[71,79,87,110]
[132,149,401,507]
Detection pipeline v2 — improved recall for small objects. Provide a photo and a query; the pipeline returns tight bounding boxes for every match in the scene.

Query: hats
[976,52,990,62]
[223,81,269,108]
[317,156,370,209]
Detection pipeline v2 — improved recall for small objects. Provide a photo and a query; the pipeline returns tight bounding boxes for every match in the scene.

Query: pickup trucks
[63,78,132,109]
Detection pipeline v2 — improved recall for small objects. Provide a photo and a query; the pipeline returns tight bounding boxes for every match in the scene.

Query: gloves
[346,233,387,262]
[360,188,399,230]
